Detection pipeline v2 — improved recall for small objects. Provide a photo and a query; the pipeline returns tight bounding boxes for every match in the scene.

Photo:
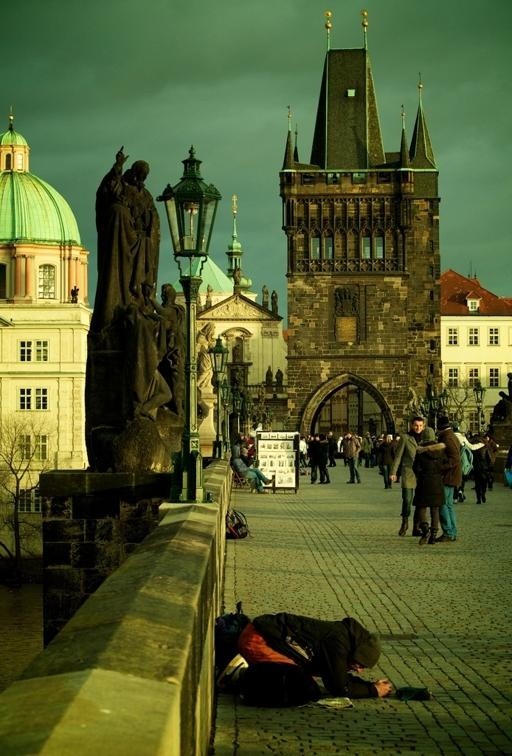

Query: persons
[216,613,391,698]
[90,146,217,420]
[231,415,512,544]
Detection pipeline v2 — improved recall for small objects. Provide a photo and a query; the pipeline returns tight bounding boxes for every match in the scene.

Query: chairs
[233,469,254,493]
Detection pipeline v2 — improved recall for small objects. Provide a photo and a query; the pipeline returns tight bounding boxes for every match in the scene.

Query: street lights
[430,392,440,437]
[205,338,245,463]
[153,143,220,503]
[438,386,454,441]
[471,379,489,441]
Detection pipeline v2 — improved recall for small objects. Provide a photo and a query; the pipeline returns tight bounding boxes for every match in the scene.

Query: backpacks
[226,508,249,539]
[459,441,474,477]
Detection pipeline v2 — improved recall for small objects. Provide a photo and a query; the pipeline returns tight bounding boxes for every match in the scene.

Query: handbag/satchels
[214,602,251,663]
[479,463,496,483]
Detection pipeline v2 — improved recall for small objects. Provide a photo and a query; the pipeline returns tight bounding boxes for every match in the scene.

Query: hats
[437,416,451,431]
[420,426,436,442]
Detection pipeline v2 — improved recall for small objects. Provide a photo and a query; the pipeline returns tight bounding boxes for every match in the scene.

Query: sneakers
[310,479,330,484]
[216,653,249,689]
[265,479,275,485]
[435,532,457,542]
[384,480,392,489]
[453,490,464,503]
[481,491,486,503]
[357,476,361,484]
[258,490,269,494]
[347,477,355,484]
[476,497,482,504]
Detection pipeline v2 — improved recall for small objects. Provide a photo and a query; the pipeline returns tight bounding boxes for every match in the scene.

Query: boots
[419,521,430,545]
[428,527,439,544]
[412,520,422,537]
[398,516,409,537]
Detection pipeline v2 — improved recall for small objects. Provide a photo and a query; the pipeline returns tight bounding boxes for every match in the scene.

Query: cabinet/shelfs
[256,430,301,494]
